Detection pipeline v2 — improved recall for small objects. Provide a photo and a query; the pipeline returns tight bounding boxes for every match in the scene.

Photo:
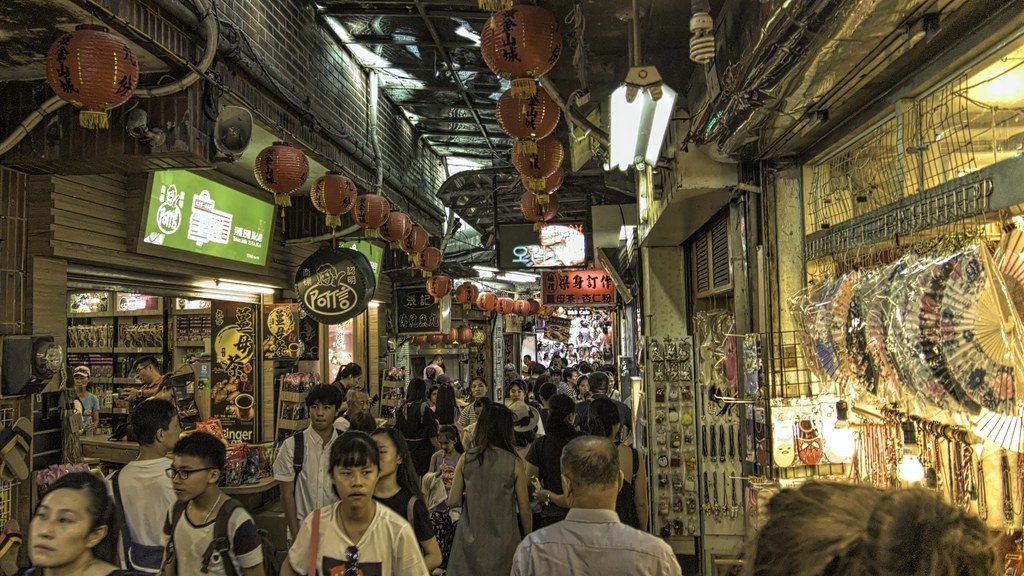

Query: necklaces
[188,494,220,524]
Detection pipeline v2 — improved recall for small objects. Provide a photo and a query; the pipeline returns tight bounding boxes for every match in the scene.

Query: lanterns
[457,281,540,317]
[426,273,451,298]
[415,327,486,352]
[310,170,357,227]
[47,24,139,130]
[350,194,441,276]
[481,4,566,223]
[255,141,309,206]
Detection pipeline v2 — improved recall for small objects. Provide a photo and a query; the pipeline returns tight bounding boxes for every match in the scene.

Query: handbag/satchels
[125,543,169,576]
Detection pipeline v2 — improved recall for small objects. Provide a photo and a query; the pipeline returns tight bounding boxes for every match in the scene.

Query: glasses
[136,363,152,373]
[165,467,215,480]
[344,545,359,576]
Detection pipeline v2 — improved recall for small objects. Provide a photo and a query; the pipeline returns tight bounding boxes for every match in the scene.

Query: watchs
[137,390,142,397]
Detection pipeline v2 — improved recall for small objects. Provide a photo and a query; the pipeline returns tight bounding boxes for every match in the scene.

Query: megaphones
[215,105,253,156]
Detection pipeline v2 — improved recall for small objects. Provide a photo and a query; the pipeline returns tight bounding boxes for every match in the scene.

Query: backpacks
[170,500,279,576]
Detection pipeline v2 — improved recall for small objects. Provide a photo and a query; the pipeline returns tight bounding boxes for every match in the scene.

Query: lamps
[496,271,538,284]
[607,0,680,174]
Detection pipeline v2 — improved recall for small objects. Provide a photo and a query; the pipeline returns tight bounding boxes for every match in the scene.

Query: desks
[219,476,279,494]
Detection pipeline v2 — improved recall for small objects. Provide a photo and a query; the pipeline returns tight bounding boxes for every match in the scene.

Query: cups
[235,394,254,418]
[289,343,299,356]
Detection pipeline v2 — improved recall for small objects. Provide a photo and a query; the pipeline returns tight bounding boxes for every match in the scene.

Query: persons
[504,342,619,468]
[121,356,164,402]
[525,395,579,525]
[396,355,488,571]
[158,432,265,576]
[273,383,344,550]
[511,436,682,576]
[447,403,533,576]
[14,471,147,576]
[74,366,101,431]
[370,427,442,572]
[278,430,429,576]
[332,361,377,432]
[533,398,648,531]
[113,398,182,576]
[754,479,997,576]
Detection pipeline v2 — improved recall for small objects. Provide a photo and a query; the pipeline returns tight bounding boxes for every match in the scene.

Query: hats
[508,400,539,432]
[437,374,450,382]
[74,366,90,378]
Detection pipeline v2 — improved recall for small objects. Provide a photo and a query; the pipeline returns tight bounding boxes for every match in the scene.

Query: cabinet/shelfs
[276,373,311,439]
[172,297,211,422]
[381,369,405,426]
[112,293,166,415]
[68,294,112,415]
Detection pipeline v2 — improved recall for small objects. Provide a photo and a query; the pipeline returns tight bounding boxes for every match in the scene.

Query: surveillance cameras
[127,110,148,138]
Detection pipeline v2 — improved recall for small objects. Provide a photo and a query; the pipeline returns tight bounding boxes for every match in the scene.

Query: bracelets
[548,491,551,502]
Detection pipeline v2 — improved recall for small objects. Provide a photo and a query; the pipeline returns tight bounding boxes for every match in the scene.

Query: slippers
[0,417,33,482]
[0,519,22,576]
